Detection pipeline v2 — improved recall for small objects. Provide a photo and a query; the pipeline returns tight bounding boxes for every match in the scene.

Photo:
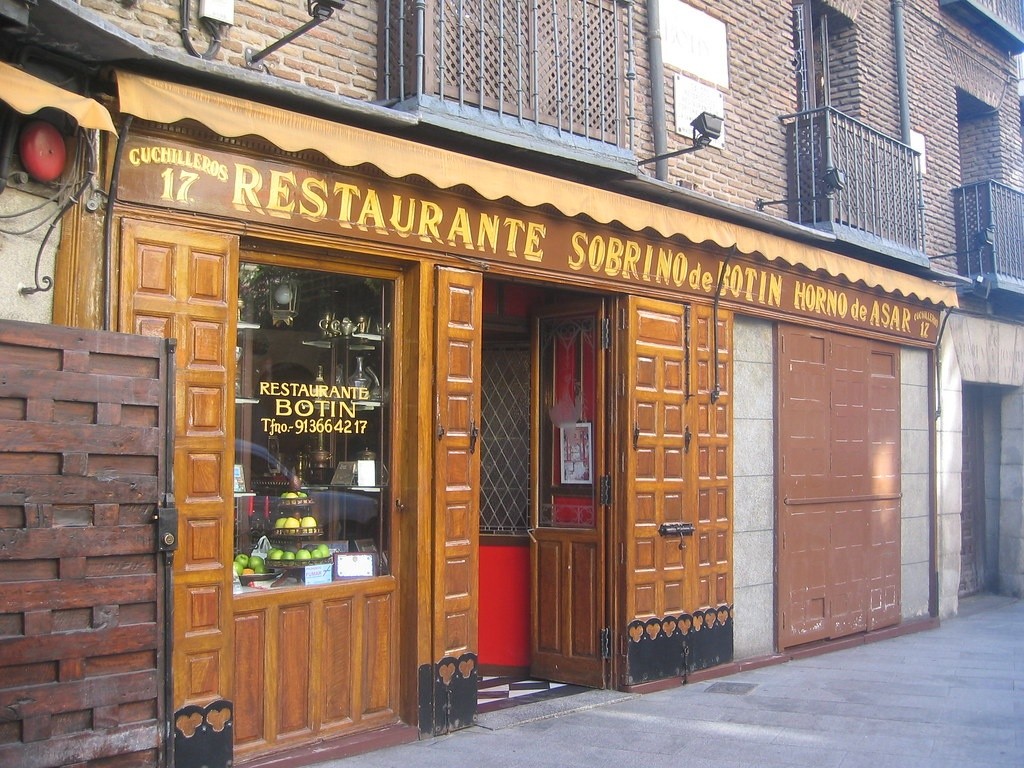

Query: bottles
[312,365,326,396]
[349,356,372,396]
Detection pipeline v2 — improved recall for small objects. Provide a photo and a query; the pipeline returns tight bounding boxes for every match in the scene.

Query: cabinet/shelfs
[302,333,383,490]
[234,321,260,496]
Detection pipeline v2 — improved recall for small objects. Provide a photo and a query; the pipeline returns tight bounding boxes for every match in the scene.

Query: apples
[233,555,266,574]
[299,493,307,498]
[268,544,328,559]
[275,518,286,528]
[301,517,316,532]
[281,493,287,497]
[284,517,299,533]
[286,493,298,498]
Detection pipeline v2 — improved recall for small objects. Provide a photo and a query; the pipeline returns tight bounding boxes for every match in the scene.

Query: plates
[240,572,281,586]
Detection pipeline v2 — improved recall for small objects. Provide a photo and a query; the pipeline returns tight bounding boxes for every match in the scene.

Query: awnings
[1,60,121,142]
[107,66,960,313]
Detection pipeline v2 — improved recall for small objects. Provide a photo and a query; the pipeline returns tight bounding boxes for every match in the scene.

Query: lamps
[757,168,845,212]
[929,227,993,259]
[638,111,725,165]
[269,267,301,327]
[245,0,346,71]
